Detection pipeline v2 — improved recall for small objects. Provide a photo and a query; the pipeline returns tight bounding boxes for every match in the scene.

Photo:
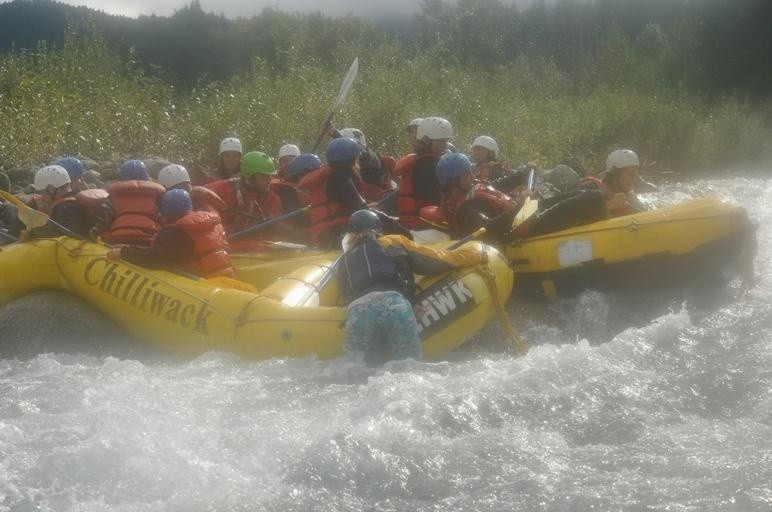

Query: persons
[193,116,639,247]
[339,210,483,369]
[0,158,235,277]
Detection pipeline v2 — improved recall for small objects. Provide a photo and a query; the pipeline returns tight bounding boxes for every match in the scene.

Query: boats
[1,226,516,375]
[500,194,755,301]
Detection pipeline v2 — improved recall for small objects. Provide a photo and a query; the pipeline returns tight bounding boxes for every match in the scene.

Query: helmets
[53,157,82,177]
[218,137,241,154]
[606,149,639,173]
[473,135,499,160]
[348,209,381,232]
[436,153,470,186]
[328,128,366,163]
[120,160,149,180]
[278,144,321,176]
[238,151,274,177]
[161,190,192,214]
[32,165,71,191]
[408,117,453,140]
[158,164,190,188]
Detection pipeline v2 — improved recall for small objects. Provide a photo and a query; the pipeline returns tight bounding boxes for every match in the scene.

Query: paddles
[0,191,113,248]
[512,168,539,232]
[176,265,258,295]
[312,57,359,154]
